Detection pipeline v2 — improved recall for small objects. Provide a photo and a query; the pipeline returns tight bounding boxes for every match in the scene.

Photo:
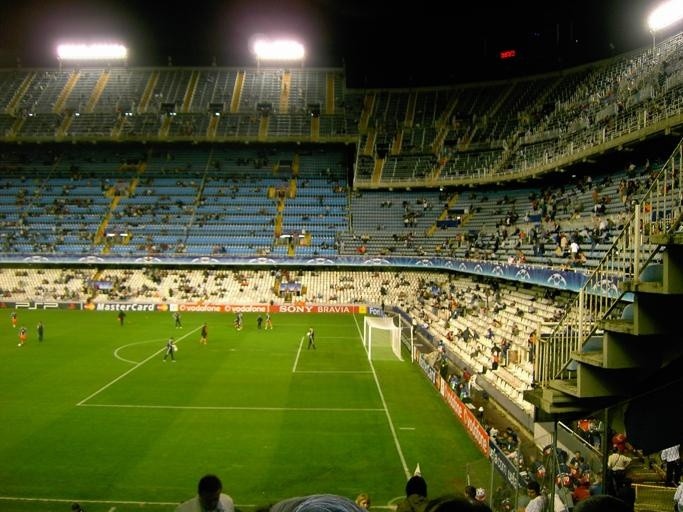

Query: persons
[119,310,125,325]
[0,30,683,303]
[307,327,315,350]
[164,337,175,362]
[233,312,272,332]
[175,412,683,511]
[175,313,182,328]
[404,299,596,414]
[10,313,44,344]
[201,321,210,343]
